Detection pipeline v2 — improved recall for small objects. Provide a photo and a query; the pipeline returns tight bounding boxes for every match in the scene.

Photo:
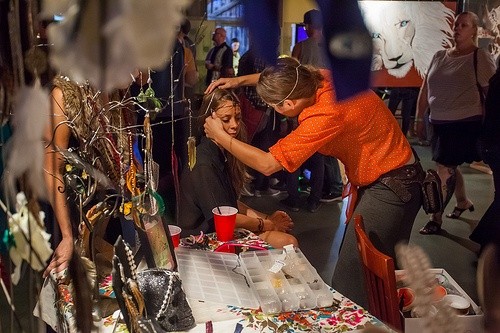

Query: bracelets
[258,218,264,232]
[414,118,423,122]
[265,216,268,219]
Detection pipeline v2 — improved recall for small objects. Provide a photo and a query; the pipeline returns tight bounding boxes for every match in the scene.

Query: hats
[299,9,320,25]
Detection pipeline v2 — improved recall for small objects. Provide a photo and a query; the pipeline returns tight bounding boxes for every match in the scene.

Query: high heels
[446,204,476,219]
[419,219,442,234]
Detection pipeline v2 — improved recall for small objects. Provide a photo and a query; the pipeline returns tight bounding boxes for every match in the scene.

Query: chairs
[354,213,402,332]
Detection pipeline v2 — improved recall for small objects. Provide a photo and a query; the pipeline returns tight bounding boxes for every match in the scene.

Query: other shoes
[280,197,302,211]
[255,186,281,197]
[320,192,343,203]
[307,201,322,212]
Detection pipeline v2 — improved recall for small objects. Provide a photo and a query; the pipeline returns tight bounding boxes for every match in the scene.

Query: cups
[212,206,238,242]
[397,285,470,318]
[167,225,181,247]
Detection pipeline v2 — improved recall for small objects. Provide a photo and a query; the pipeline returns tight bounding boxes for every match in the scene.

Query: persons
[119,17,200,208]
[413,10,496,235]
[176,86,299,252]
[203,56,426,310]
[239,9,345,213]
[230,39,241,76]
[34,69,129,333]
[204,28,233,86]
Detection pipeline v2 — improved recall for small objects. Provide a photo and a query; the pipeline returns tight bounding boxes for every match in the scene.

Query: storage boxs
[394,268,485,333]
[175,246,333,315]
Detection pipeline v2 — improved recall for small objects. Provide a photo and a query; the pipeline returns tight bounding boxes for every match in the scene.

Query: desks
[32,227,397,333]
